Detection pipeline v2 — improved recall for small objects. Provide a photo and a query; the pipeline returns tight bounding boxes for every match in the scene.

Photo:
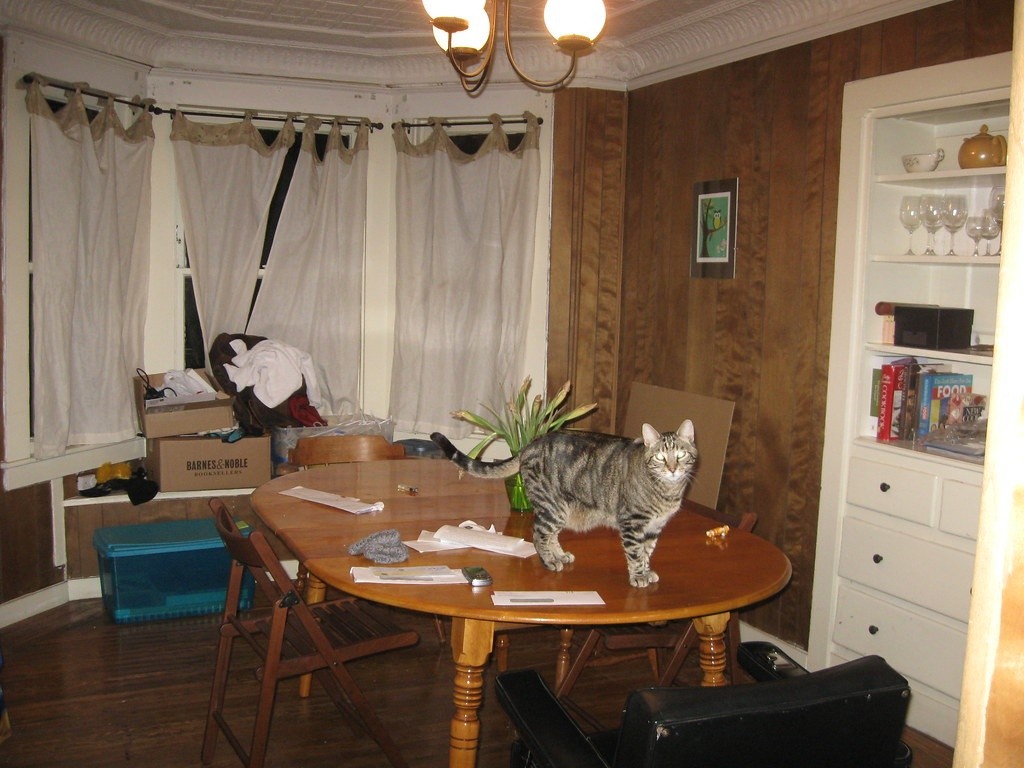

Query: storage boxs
[893,306,974,350]
[92,367,443,625]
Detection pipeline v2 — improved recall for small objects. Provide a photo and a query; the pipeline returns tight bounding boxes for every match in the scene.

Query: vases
[503,474,533,513]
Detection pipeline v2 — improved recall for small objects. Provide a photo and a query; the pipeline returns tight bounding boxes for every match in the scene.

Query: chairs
[494,641,913,768]
[554,496,758,729]
[202,435,448,768]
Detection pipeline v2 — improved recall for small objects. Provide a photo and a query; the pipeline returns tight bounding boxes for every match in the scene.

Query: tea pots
[958,124,1008,169]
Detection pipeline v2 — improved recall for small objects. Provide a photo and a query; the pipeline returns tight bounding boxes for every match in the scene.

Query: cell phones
[462,567,492,586]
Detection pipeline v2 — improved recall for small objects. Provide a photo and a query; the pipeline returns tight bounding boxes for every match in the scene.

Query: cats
[429,418,698,589]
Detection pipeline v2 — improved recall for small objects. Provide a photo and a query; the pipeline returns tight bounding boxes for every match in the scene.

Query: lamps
[421,0,607,93]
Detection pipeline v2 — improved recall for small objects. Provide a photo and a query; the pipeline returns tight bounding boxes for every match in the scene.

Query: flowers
[451,374,599,507]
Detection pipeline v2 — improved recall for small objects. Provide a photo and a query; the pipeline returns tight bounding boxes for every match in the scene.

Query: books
[867,364,988,463]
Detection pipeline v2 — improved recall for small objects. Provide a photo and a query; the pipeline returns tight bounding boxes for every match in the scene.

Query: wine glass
[980,209,1000,257]
[965,216,983,257]
[988,185,1005,257]
[942,194,968,257]
[919,194,944,256]
[899,195,922,256]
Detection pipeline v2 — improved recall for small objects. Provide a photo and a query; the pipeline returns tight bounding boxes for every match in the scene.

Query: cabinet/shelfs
[805,49,1015,750]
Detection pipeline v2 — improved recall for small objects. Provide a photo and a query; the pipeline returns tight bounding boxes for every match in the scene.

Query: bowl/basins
[901,149,945,173]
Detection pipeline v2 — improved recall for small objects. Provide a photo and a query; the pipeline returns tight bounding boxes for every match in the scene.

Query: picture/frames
[690,176,738,279]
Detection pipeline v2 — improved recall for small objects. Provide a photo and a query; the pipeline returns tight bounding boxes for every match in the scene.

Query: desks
[247,461,793,768]
